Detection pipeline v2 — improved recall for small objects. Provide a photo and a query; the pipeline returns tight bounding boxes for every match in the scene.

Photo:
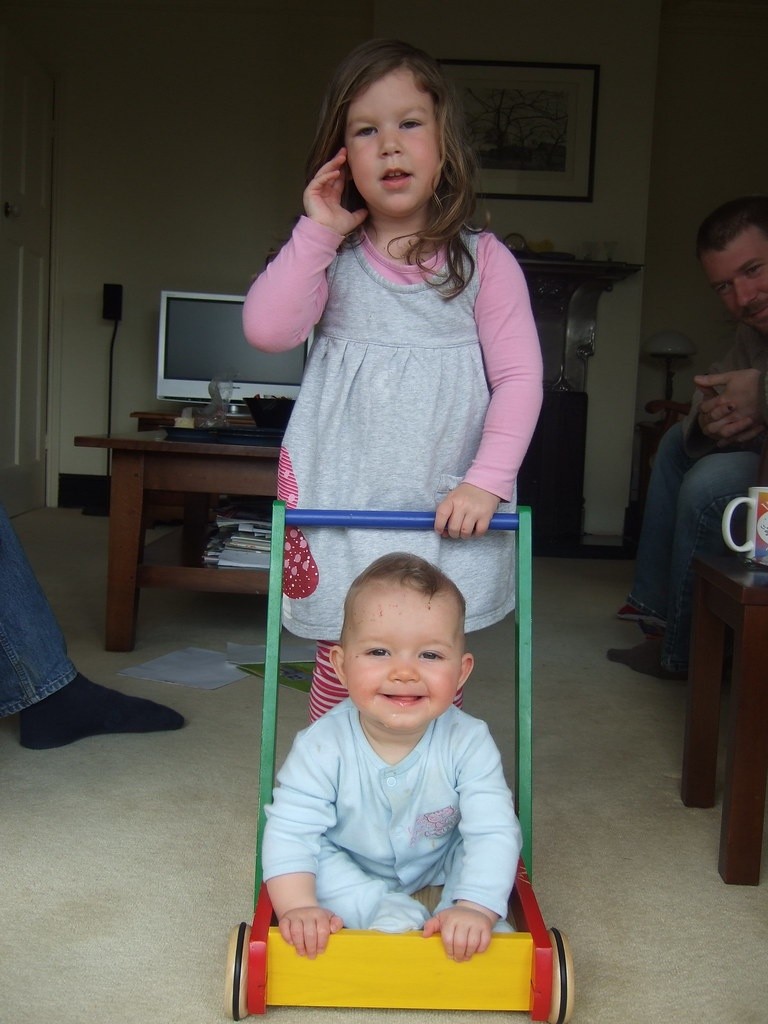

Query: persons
[241,36,544,729]
[0,496,185,750]
[606,195,768,678]
[261,551,524,963]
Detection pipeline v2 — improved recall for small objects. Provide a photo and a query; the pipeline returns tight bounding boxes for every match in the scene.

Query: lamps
[649,330,697,428]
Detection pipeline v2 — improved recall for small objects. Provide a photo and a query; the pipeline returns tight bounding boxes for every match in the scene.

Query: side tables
[682,558,768,886]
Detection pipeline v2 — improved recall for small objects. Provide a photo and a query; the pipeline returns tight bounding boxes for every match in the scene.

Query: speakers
[102,283,123,320]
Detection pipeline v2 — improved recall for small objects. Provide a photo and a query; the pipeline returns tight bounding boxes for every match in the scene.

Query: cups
[722,485,768,566]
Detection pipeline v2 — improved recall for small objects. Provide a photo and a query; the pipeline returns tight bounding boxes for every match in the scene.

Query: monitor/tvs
[154,290,314,420]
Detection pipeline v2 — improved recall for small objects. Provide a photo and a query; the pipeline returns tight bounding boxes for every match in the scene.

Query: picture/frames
[435,58,600,202]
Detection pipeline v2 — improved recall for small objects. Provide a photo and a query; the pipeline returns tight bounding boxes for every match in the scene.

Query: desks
[74,430,282,651]
[637,419,661,515]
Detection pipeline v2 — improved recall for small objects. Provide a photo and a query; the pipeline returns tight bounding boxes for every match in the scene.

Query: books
[199,503,272,572]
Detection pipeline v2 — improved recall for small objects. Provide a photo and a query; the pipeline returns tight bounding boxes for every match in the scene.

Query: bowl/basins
[243,398,295,429]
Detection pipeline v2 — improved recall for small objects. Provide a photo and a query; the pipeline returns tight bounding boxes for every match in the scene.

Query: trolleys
[224,499,574,1024]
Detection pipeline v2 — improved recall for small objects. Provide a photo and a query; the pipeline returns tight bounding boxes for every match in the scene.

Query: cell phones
[697,373,719,400]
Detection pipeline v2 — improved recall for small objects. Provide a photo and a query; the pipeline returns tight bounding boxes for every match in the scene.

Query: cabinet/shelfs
[130,411,256,529]
